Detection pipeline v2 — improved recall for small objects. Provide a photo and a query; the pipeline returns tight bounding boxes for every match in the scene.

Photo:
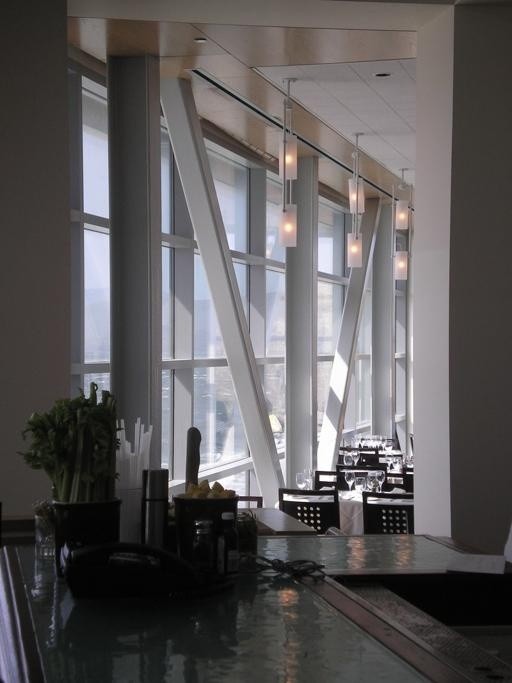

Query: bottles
[192,510,241,573]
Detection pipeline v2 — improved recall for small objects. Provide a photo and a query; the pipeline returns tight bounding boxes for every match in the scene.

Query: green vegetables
[16,382,123,503]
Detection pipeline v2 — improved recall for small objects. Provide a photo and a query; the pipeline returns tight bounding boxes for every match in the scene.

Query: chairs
[233,438,415,534]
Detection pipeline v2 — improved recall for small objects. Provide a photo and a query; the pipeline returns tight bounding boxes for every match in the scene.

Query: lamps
[277,77,410,280]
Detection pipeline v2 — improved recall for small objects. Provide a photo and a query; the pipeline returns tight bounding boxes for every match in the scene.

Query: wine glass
[294,433,404,500]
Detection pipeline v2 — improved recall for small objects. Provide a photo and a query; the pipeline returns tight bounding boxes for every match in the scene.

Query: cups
[33,511,60,565]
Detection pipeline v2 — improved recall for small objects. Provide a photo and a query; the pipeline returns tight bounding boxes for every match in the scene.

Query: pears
[186,479,235,500]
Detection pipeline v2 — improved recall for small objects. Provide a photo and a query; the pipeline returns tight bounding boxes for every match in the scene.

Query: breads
[185,427,201,493]
